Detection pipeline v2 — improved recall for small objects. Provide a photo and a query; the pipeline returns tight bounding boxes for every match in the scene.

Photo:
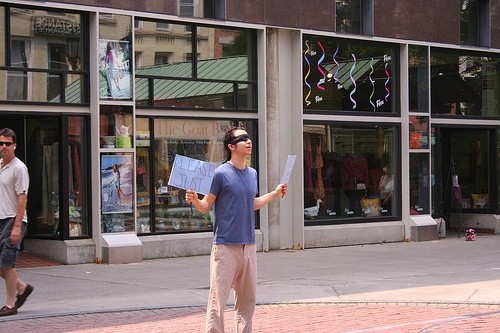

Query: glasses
[0,142,14,146]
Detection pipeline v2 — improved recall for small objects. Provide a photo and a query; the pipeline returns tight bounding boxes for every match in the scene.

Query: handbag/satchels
[360,197,382,217]
[471,194,488,208]
[353,177,366,191]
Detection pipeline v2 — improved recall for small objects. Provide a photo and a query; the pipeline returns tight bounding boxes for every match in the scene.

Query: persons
[0,128,34,316]
[105,42,123,91]
[377,166,395,217]
[185,126,288,333]
[106,164,126,200]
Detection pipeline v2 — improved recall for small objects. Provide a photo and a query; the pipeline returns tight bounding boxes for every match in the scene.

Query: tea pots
[115,125,133,137]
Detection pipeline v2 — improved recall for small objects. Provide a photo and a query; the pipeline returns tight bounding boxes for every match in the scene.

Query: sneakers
[15,283,33,309]
[0,306,17,315]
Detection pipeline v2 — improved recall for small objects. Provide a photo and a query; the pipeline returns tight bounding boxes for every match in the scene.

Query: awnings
[48,55,385,103]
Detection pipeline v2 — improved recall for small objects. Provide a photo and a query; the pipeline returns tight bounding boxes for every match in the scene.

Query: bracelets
[13,224,21,228]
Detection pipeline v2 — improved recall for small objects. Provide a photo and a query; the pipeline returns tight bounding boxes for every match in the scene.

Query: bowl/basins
[100,136,115,144]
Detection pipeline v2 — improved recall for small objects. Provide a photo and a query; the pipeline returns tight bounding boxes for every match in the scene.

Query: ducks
[304,199,324,220]
